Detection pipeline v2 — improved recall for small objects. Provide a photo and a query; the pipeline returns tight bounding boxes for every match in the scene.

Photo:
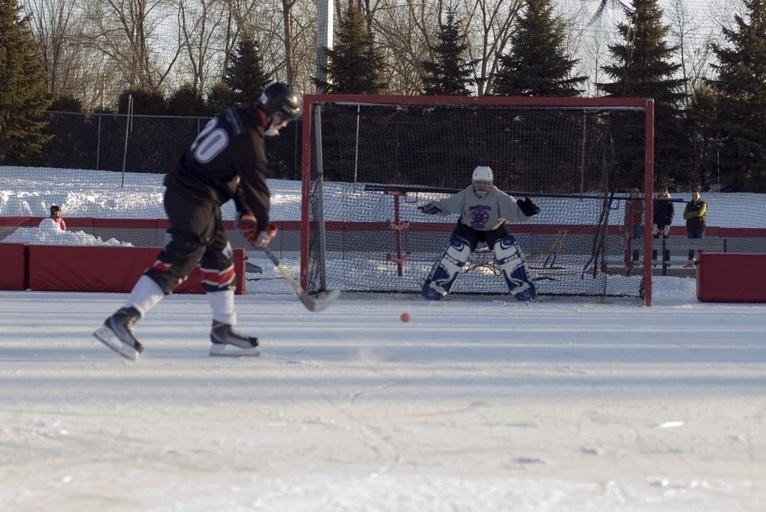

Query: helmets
[254,81,305,120]
[471,164,494,186]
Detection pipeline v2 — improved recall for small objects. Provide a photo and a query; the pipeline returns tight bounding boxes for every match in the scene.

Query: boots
[102,307,145,352]
[209,319,259,348]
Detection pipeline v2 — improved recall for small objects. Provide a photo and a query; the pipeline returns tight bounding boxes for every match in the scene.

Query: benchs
[621,237,727,275]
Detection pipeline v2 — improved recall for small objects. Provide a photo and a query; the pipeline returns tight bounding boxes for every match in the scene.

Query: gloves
[516,197,541,217]
[416,203,440,216]
[237,211,277,250]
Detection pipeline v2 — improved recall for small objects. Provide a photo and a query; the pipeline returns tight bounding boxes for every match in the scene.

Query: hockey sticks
[264,246,342,312]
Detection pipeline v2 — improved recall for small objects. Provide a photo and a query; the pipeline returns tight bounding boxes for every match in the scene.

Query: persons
[415,166,539,303]
[682,190,709,268]
[651,186,676,267]
[622,186,644,265]
[102,80,300,354]
[47,205,65,229]
